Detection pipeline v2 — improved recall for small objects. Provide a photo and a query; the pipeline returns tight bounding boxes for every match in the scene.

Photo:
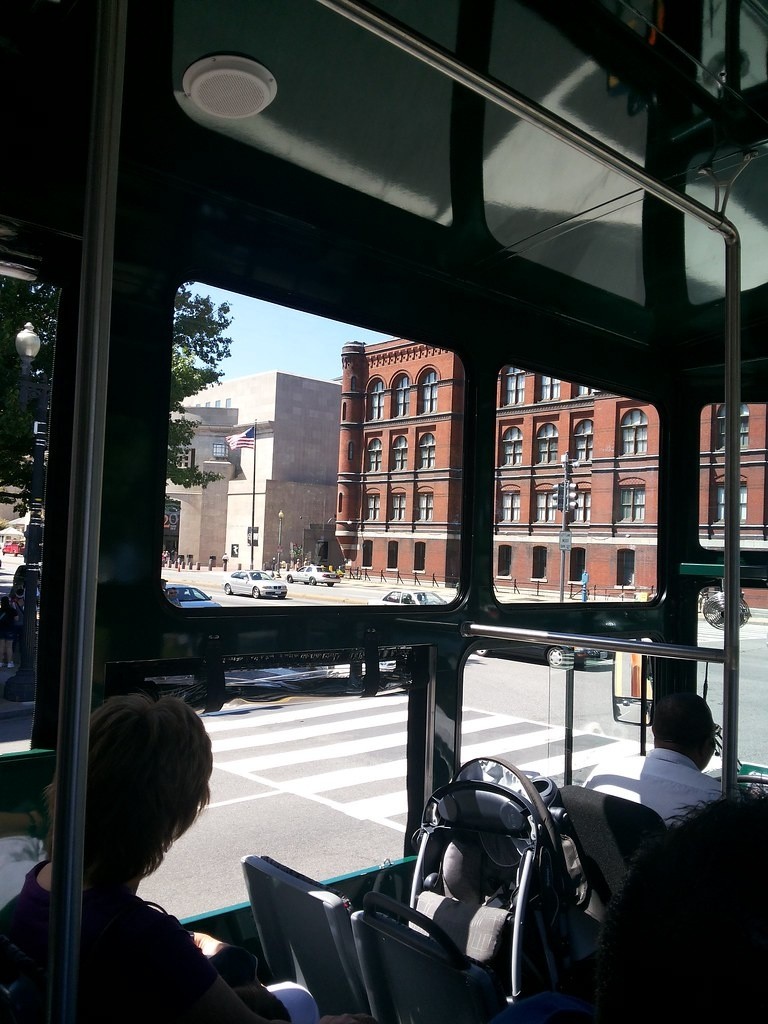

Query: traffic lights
[553,483,563,511]
[566,480,579,510]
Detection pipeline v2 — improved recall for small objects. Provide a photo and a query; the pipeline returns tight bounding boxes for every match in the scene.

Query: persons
[0,793,50,911]
[579,692,722,830]
[0,686,382,1024]
[487,775,768,1024]
[2,576,40,669]
[162,544,240,572]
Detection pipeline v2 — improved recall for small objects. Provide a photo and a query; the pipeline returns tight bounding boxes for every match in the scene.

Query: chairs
[558,783,666,929]
[232,850,502,1024]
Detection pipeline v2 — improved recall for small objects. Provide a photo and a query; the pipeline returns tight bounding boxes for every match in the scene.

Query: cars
[222,571,287,599]
[3,540,26,557]
[284,566,341,587]
[367,589,450,605]
[163,584,222,608]
[473,645,615,670]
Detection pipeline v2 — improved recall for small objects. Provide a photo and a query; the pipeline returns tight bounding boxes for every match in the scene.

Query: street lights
[276,510,284,577]
[2,320,52,702]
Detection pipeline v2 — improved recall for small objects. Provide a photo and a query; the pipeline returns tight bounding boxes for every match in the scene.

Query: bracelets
[25,810,37,838]
